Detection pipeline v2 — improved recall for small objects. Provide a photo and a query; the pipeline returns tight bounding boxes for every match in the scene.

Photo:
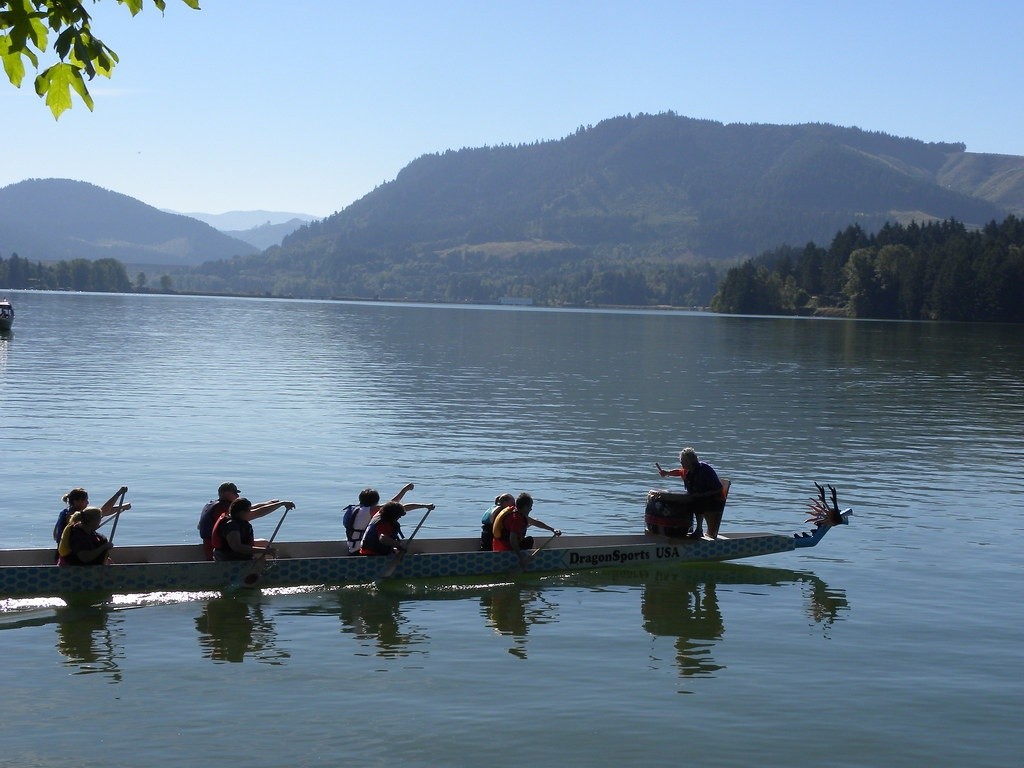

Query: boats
[2,480,853,599]
[0,298,14,331]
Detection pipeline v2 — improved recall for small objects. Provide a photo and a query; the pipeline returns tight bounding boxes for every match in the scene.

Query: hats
[217,482,241,493]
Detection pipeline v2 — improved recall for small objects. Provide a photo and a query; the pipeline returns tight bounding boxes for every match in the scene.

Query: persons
[359,498,437,556]
[490,490,562,572]
[660,443,726,538]
[50,487,131,563]
[342,481,414,555]
[200,480,280,559]
[56,484,129,567]
[480,491,517,552]
[210,500,296,561]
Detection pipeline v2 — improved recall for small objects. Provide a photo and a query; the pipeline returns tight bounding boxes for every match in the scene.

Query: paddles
[100,490,125,576]
[512,532,558,576]
[377,508,432,579]
[241,508,289,590]
[94,509,127,533]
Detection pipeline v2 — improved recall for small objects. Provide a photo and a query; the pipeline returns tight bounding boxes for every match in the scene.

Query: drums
[644,488,692,539]
